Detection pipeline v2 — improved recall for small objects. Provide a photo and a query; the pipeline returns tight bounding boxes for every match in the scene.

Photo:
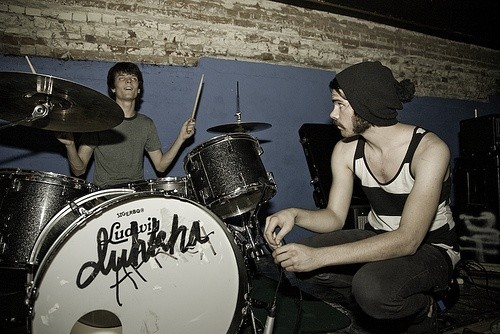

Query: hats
[335,59,415,126]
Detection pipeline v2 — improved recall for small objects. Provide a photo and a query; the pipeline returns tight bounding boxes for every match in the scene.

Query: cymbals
[205,121,273,135]
[0,69,126,134]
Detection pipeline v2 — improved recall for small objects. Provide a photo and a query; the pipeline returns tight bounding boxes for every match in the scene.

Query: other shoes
[407,300,437,334]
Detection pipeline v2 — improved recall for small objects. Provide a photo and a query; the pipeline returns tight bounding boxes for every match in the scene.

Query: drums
[0,167,105,279]
[109,175,197,204]
[23,185,252,333]
[182,133,279,220]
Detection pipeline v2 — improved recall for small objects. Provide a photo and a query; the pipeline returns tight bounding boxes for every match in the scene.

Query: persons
[264,62,460,334]
[55,62,196,186]
